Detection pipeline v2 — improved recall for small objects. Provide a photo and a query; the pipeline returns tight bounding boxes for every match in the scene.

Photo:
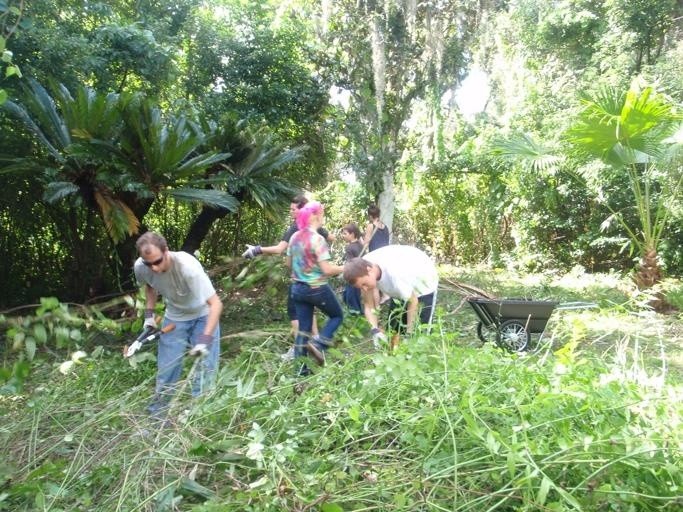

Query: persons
[242,195,439,376]
[134,232,223,418]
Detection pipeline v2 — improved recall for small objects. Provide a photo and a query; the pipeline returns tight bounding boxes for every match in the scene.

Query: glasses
[141,256,165,267]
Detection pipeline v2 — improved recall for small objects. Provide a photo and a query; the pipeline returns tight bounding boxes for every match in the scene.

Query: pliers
[123,316,176,359]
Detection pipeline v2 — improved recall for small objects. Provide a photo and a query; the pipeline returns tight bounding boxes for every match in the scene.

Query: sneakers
[147,402,163,413]
[307,336,326,364]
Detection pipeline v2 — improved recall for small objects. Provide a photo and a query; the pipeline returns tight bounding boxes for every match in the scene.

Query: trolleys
[464,293,599,352]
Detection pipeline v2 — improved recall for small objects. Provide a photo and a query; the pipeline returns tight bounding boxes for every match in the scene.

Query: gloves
[371,327,387,349]
[242,244,263,260]
[143,309,159,330]
[190,334,214,358]
